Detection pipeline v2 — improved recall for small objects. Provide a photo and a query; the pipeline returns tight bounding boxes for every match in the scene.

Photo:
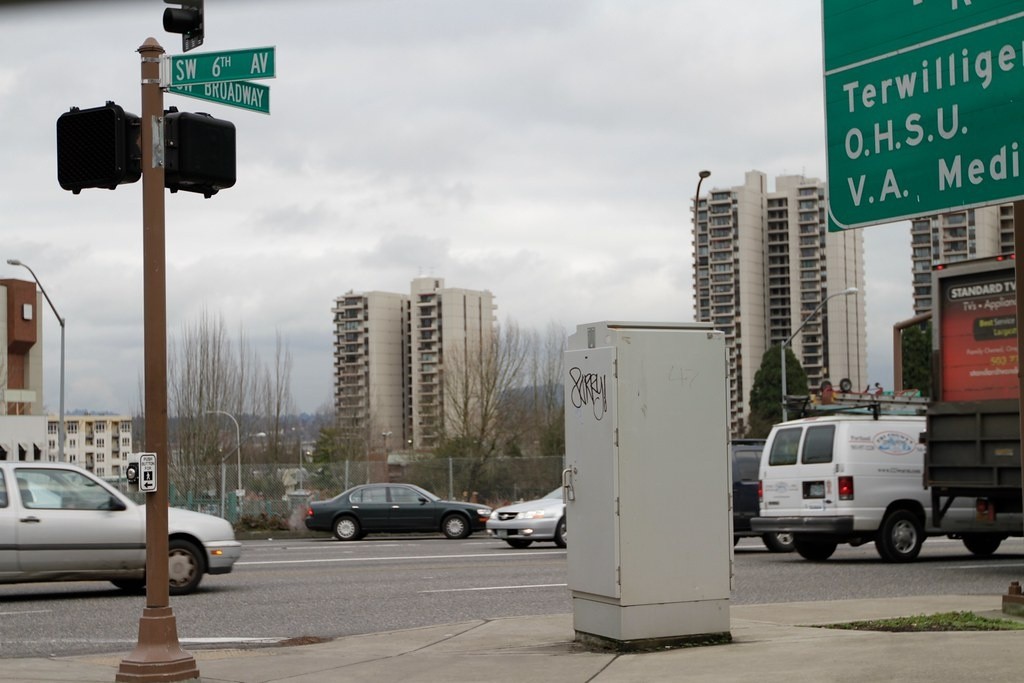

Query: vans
[750,404,1002,562]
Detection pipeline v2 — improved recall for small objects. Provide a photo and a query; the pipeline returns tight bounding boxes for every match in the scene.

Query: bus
[917,251,1024,545]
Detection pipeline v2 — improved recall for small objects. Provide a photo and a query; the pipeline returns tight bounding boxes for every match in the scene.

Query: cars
[725,436,793,553]
[302,479,495,540]
[484,485,570,547]
[0,462,242,596]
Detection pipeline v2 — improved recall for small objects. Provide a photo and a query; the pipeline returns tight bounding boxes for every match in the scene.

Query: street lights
[694,171,711,321]
[202,409,245,522]
[7,256,68,461]
[780,287,858,422]
